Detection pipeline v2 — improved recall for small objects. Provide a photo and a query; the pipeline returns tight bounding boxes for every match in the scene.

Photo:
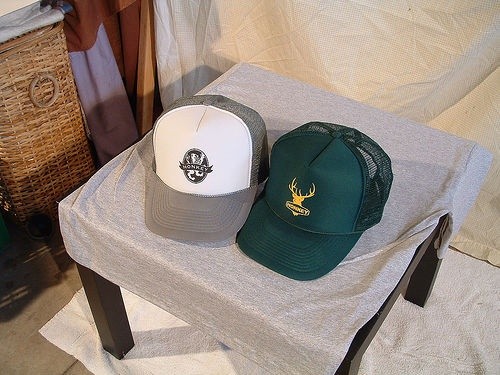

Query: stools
[56,63,476,375]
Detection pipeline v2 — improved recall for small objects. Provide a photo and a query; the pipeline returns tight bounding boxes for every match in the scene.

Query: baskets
[0,17,101,231]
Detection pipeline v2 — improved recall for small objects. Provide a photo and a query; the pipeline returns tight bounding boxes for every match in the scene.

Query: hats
[143,93,269,243]
[236,121,394,282]
